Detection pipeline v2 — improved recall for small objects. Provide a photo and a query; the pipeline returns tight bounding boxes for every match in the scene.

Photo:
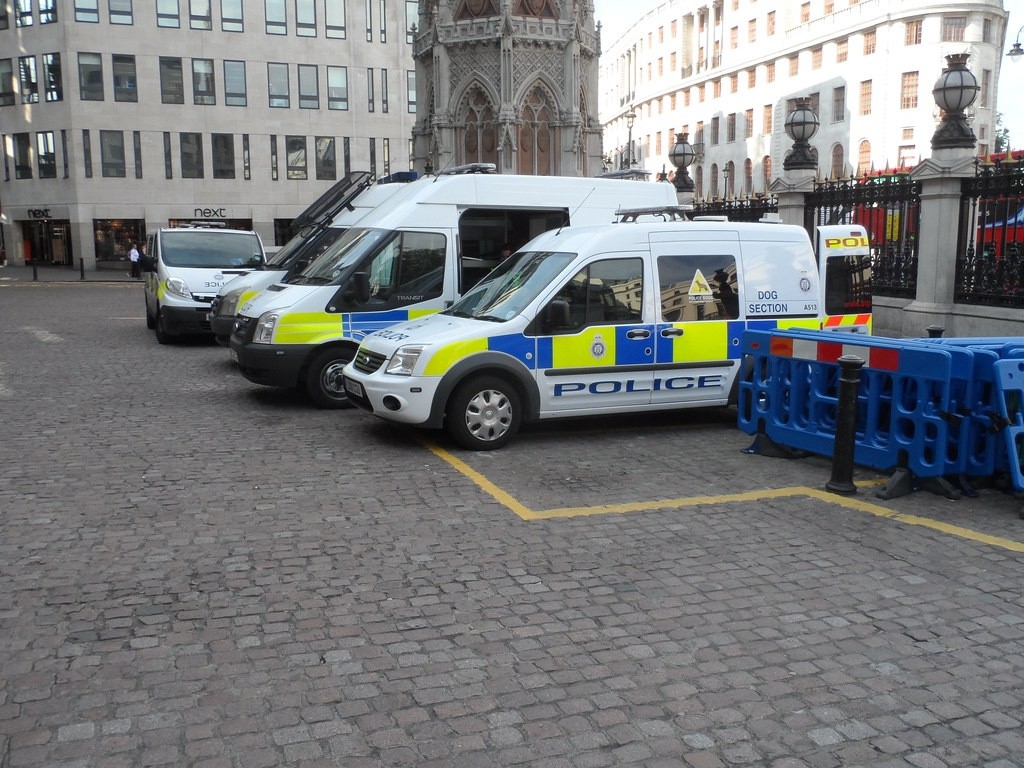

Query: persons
[493,243,515,269]
[127,242,140,277]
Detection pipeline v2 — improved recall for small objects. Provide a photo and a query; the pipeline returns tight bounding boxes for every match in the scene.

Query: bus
[851,148,1024,273]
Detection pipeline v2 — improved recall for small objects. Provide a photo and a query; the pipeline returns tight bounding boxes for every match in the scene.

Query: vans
[138,222,268,346]
[228,163,684,411]
[207,170,420,349]
[339,205,873,451]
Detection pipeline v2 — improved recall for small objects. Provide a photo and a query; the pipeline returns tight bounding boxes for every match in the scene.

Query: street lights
[620,149,638,171]
[722,166,731,199]
[624,113,637,169]
[602,158,614,173]
